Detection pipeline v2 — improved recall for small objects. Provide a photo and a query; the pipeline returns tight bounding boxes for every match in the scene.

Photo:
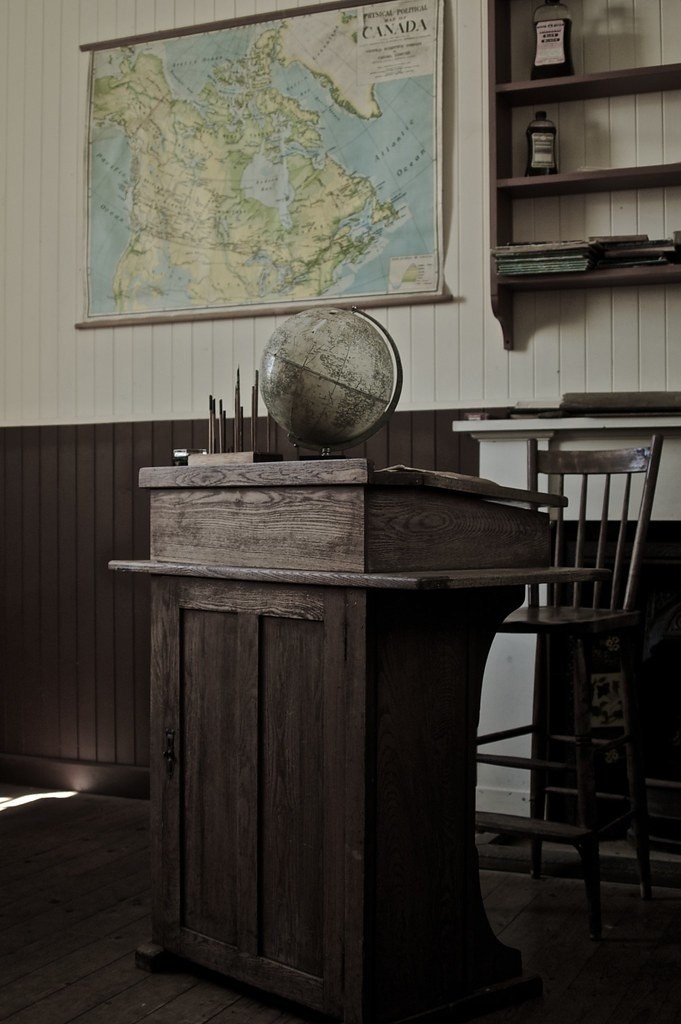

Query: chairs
[476,432,670,939]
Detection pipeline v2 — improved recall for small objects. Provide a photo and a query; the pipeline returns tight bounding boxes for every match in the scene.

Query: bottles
[530,0,574,80]
[525,110,557,177]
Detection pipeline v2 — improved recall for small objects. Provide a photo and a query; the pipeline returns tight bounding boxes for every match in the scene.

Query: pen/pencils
[208,364,263,452]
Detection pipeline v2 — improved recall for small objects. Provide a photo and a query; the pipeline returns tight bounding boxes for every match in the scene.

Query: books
[492,230,680,274]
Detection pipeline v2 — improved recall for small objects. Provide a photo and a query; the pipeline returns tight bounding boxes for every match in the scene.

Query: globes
[257,301,404,458]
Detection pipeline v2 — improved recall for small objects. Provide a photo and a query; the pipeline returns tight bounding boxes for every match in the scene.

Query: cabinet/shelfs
[488,0,681,351]
